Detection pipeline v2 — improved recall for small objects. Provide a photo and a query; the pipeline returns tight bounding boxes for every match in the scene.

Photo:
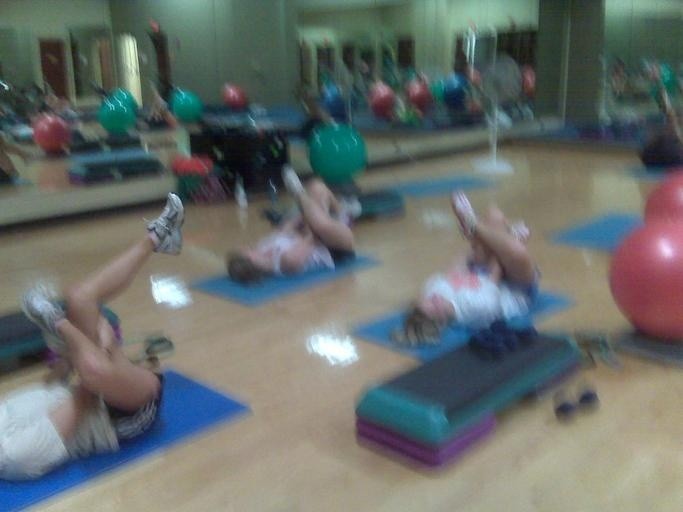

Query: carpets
[66,146,158,163]
[549,211,652,260]
[352,272,577,365]
[375,175,493,195]
[0,369,254,512]
[187,252,380,308]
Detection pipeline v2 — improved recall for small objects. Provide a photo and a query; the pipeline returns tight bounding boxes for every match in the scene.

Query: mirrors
[0,1,571,234]
[553,1,681,136]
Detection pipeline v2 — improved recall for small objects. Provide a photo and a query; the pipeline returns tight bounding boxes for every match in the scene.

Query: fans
[471,51,522,180]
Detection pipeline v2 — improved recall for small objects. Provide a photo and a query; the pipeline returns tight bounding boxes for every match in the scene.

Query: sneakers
[23,288,68,342]
[450,190,477,237]
[283,166,304,197]
[148,192,184,256]
[512,219,530,242]
[338,199,361,227]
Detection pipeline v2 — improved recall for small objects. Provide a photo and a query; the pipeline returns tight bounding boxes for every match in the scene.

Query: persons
[0,14,537,184]
[639,67,682,165]
[222,163,360,285]
[386,188,545,350]
[1,189,185,483]
